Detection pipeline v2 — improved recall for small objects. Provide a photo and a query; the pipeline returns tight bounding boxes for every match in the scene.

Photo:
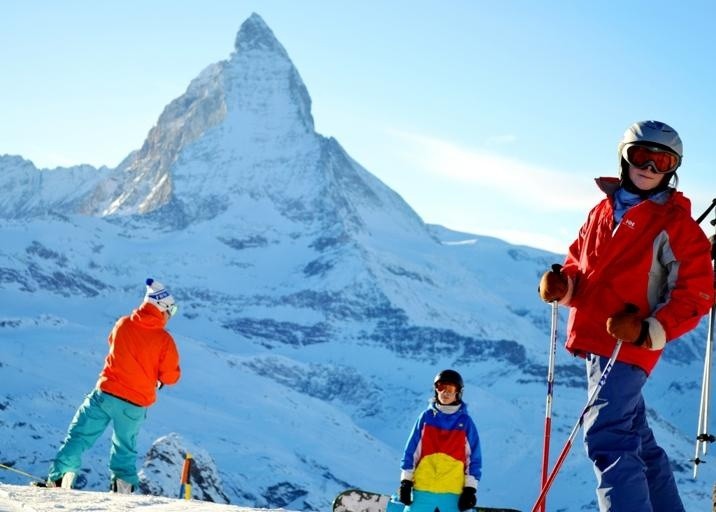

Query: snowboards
[334,491,521,512]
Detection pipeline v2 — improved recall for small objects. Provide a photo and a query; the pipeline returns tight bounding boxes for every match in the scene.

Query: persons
[31,279,180,494]
[400,370,482,512]
[537,119,716,511]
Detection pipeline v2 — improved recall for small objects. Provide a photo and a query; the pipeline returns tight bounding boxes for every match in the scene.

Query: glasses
[622,144,679,174]
[437,384,456,392]
[158,302,177,316]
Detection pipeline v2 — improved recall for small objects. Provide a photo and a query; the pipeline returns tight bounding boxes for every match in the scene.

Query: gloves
[540,270,573,305]
[607,311,649,346]
[459,487,476,511]
[400,480,413,505]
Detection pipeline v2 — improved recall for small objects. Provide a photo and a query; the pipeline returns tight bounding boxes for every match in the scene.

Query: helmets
[618,121,683,166]
[434,370,463,390]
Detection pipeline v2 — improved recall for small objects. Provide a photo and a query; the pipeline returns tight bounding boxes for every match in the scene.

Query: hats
[144,278,175,311]
